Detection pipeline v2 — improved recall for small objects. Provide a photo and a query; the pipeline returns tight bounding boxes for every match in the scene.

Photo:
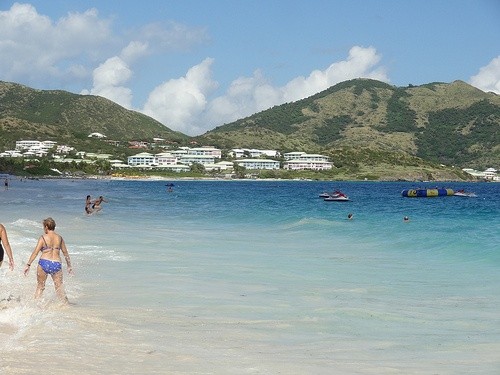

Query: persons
[5,178,8,190]
[0,224,14,271]
[85,195,93,213]
[24,217,72,302]
[348,213,352,218]
[92,196,108,211]
[404,216,408,220]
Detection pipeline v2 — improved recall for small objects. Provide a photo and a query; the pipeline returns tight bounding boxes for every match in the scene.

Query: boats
[318,193,330,198]
[402,188,455,198]
[324,194,351,201]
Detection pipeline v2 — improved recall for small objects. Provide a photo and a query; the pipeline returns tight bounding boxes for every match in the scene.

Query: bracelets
[27,263,30,266]
